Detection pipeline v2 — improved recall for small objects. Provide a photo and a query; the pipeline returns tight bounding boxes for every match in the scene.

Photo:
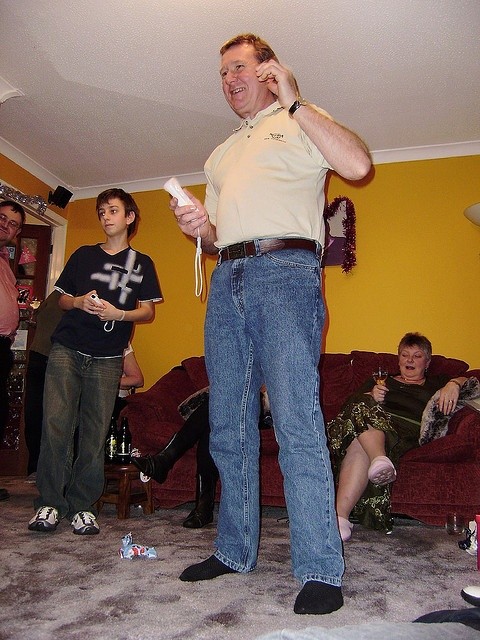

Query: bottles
[105,415,118,463]
[115,416,132,466]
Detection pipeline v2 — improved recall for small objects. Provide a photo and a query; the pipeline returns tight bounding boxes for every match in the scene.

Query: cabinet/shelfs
[3,225,52,328]
[2,350,47,478]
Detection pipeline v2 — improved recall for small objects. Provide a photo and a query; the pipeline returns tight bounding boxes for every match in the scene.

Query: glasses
[0,215,18,229]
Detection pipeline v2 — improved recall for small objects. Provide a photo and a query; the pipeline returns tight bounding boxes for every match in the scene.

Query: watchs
[288,96,308,117]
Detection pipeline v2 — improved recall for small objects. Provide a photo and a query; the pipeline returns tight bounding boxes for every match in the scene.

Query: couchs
[117,351,480,535]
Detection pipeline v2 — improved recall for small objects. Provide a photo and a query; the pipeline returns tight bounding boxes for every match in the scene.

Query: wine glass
[371,365,388,407]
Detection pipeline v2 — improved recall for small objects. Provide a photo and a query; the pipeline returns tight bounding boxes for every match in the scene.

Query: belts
[217,238,322,263]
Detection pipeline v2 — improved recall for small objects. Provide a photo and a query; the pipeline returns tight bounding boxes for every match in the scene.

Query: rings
[449,400,453,403]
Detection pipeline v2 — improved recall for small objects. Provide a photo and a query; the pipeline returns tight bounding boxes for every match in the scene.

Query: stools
[97,461,154,519]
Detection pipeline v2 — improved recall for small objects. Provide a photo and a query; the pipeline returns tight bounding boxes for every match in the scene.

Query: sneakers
[28,505,61,531]
[71,511,100,535]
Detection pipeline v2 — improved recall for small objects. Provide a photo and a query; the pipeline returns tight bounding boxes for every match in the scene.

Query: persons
[28,188,164,534]
[24,289,70,484]
[130,389,271,529]
[0,201,25,500]
[112,339,145,419]
[168,33,372,615]
[335,333,468,541]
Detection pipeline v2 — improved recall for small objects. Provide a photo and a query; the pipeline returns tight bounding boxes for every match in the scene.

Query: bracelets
[448,380,462,391]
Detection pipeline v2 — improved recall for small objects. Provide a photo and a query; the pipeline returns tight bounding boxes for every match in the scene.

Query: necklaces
[401,377,425,381]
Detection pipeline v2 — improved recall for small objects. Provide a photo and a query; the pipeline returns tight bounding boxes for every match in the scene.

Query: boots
[183,471,218,529]
[129,432,194,484]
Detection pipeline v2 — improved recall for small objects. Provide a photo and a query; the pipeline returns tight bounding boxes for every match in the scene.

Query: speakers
[52,185,73,210]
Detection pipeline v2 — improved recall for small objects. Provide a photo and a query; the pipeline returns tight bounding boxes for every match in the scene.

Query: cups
[446,512,470,535]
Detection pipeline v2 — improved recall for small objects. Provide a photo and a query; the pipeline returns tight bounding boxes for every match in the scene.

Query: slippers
[368,456,397,487]
[337,516,354,542]
